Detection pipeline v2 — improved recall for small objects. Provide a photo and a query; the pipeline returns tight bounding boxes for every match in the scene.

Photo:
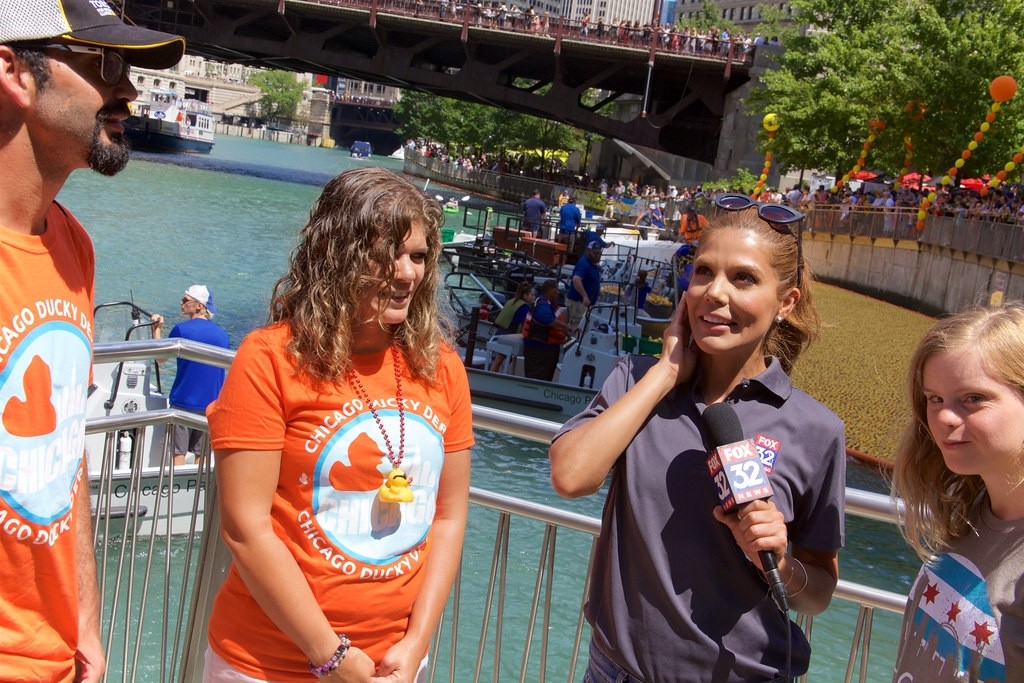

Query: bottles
[583,371,590,388]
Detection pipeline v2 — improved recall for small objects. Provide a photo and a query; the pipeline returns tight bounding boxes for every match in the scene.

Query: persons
[549,192,846,683]
[491,190,710,380]
[580,12,590,41]
[0,0,187,683]
[176,112,191,139]
[407,0,549,38]
[150,284,231,466]
[202,167,474,683]
[403,139,533,179]
[599,182,1024,242]
[890,302,1024,683]
[597,18,782,63]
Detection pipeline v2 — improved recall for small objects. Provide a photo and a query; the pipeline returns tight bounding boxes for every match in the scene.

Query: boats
[439,201,684,424]
[351,141,372,159]
[122,89,214,153]
[387,147,405,160]
[84,300,217,535]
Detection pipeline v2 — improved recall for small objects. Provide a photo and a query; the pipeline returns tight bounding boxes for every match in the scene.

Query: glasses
[182,298,193,303]
[715,192,805,288]
[10,37,132,85]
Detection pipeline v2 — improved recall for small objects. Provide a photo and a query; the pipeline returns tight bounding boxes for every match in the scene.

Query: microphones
[700,403,789,612]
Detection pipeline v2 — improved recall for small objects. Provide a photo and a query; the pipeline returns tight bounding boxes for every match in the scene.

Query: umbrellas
[902,172,930,185]
[851,170,878,182]
[962,174,991,190]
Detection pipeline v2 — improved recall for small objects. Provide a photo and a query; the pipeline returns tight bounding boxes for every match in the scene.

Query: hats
[650,204,656,210]
[597,224,604,230]
[184,284,217,315]
[0,0,185,71]
[588,241,602,250]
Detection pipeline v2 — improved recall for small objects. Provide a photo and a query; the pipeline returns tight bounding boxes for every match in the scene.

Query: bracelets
[784,553,808,599]
[307,634,352,678]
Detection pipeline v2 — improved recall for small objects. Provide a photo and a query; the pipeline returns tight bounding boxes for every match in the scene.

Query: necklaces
[347,335,414,503]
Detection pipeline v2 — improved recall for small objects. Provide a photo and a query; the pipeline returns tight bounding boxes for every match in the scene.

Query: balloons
[893,99,926,182]
[750,113,782,199]
[831,120,886,189]
[977,154,1024,198]
[916,75,1016,234]
